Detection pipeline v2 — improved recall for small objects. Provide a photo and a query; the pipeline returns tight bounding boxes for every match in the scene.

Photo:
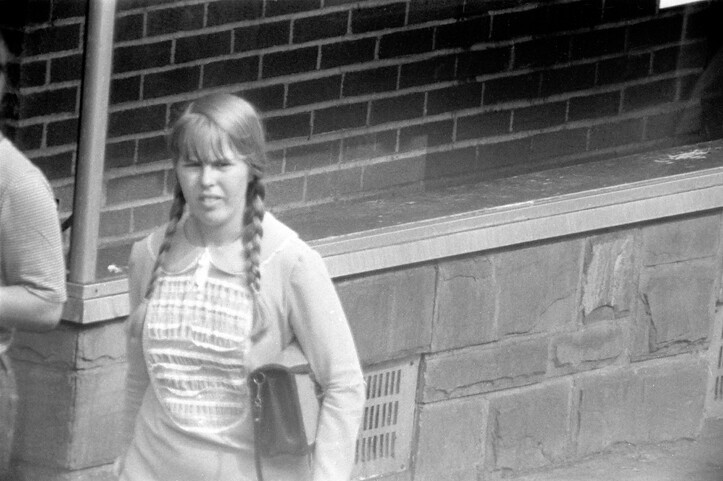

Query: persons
[0,42,65,481]
[112,92,366,481]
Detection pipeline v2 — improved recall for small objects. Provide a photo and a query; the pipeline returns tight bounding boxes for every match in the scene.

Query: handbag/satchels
[247,340,323,481]
